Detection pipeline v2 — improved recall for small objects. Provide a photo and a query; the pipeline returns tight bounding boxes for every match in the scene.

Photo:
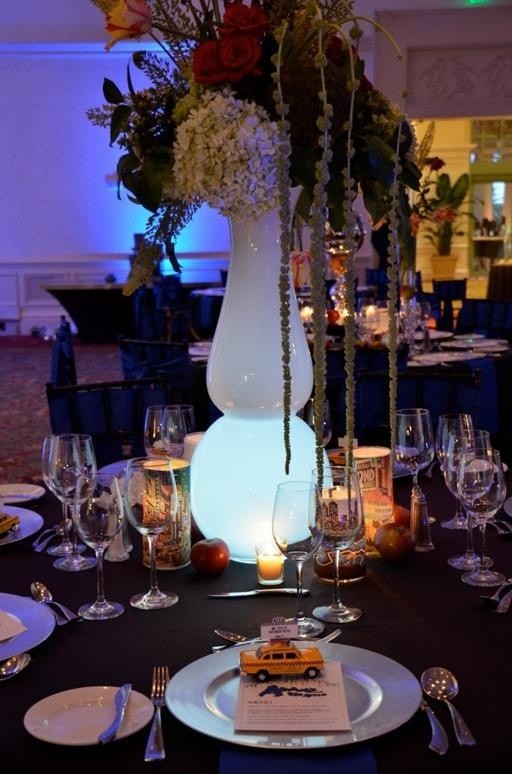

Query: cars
[441,341,509,346]
[256,537,287,584]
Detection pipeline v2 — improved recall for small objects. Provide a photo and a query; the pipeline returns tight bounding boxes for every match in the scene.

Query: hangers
[421,668,474,747]
[215,629,319,642]
[36,519,73,550]
[1,656,30,679]
[30,581,82,626]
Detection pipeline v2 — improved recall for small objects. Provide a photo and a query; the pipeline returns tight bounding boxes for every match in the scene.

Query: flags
[190,120,334,567]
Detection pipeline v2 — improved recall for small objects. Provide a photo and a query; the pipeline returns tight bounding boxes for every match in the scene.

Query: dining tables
[424,171,470,280]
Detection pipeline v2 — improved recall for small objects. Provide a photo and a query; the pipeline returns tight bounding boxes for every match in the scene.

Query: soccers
[372,523,415,563]
[189,538,229,577]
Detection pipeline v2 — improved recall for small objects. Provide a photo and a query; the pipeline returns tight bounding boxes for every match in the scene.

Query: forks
[144,665,168,761]
[213,629,343,647]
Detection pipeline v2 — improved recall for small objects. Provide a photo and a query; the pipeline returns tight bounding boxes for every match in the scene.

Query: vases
[85,0,436,518]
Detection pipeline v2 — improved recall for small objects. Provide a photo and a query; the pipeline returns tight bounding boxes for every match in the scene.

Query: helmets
[1,507,41,545]
[1,484,44,503]
[413,353,486,361]
[0,592,56,661]
[24,686,153,745]
[165,640,422,747]
[402,331,453,338]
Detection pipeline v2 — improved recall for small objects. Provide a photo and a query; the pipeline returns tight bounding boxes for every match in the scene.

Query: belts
[40,435,94,554]
[73,473,125,618]
[398,304,422,356]
[146,405,196,460]
[52,432,96,570]
[274,484,324,638]
[437,415,507,587]
[125,460,177,608]
[357,297,378,347]
[394,408,435,521]
[310,468,363,622]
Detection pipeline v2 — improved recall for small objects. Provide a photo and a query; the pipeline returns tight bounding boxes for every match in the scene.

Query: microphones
[432,277,467,331]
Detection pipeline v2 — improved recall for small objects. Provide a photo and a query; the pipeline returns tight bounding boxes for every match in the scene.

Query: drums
[98,684,132,745]
[209,589,311,597]
[419,699,448,756]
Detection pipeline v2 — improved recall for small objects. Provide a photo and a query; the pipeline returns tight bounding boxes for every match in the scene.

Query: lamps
[46,313,146,388]
[44,372,147,479]
